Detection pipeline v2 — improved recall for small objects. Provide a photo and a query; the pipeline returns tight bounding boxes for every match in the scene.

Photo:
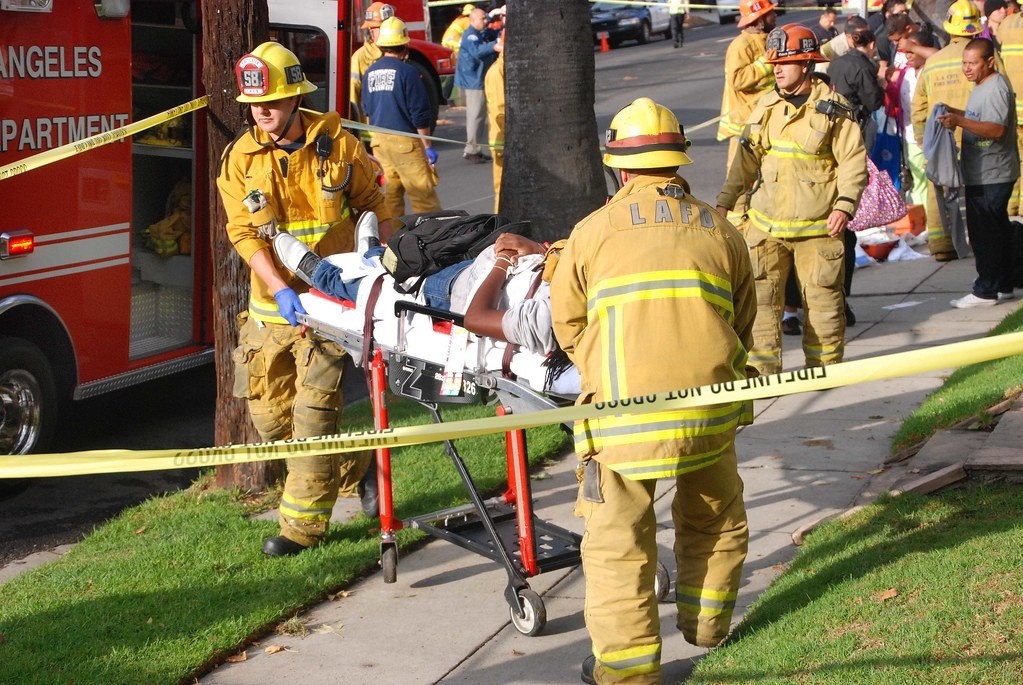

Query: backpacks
[379,209,532,294]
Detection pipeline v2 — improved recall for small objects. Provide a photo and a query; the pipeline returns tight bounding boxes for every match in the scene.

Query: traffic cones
[599,33,610,52]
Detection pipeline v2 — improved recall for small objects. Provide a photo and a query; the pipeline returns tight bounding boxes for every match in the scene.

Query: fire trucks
[0,0,455,500]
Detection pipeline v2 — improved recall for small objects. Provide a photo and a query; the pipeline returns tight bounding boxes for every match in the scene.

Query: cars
[590,0,674,44]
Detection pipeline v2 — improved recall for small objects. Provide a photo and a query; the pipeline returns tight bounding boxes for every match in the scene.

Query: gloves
[274,287,308,327]
[426,147,438,165]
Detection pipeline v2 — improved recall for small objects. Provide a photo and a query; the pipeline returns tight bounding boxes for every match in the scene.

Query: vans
[715,0,786,24]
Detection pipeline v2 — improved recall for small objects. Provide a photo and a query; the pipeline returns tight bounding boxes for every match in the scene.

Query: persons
[351,2,441,224]
[272,211,572,395]
[667,0,690,48]
[550,97,757,685]
[716,22,868,376]
[813,0,941,248]
[716,0,780,178]
[441,3,506,203]
[215,41,398,557]
[909,0,1023,307]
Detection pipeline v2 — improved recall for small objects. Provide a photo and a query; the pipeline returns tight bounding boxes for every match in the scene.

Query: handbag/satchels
[845,61,910,231]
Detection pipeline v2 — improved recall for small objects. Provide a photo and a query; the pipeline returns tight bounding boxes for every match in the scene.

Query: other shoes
[581,655,597,685]
[782,316,804,335]
[998,292,1015,300]
[272,231,322,287]
[260,535,308,556]
[935,251,958,261]
[357,449,380,518]
[353,209,379,254]
[463,152,492,164]
[846,304,856,326]
[950,293,998,308]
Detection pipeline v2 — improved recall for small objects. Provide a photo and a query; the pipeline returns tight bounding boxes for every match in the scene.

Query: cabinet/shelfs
[131,21,195,284]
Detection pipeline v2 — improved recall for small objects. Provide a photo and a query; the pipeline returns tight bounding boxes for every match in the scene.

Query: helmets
[494,27,504,53]
[942,0,984,36]
[376,16,410,47]
[737,0,778,28]
[602,97,694,169]
[236,41,318,103]
[360,2,394,29]
[764,24,831,63]
[462,4,475,16]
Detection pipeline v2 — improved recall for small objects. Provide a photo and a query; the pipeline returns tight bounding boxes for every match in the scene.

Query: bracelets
[493,253,513,273]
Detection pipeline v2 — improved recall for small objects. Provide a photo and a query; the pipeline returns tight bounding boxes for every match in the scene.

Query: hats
[984,0,1008,17]
